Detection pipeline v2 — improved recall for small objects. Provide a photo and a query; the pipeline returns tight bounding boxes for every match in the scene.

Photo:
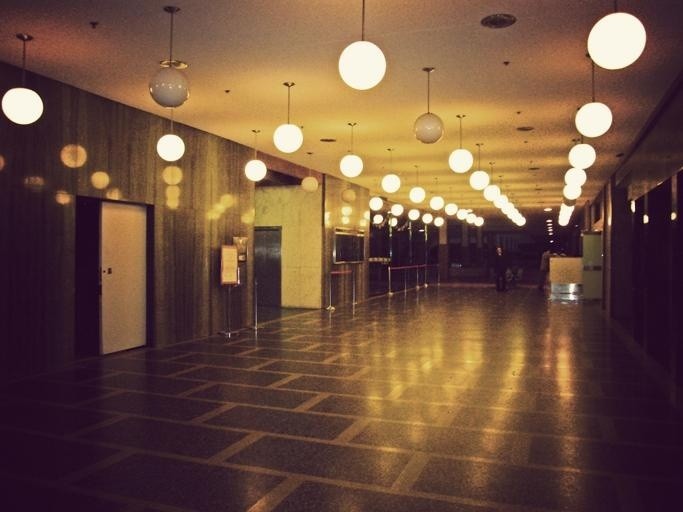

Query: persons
[493,247,509,292]
[534,248,557,292]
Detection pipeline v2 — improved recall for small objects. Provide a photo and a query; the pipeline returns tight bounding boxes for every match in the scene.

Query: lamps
[0,99,257,225]
[148,5,191,107]
[414,67,443,144]
